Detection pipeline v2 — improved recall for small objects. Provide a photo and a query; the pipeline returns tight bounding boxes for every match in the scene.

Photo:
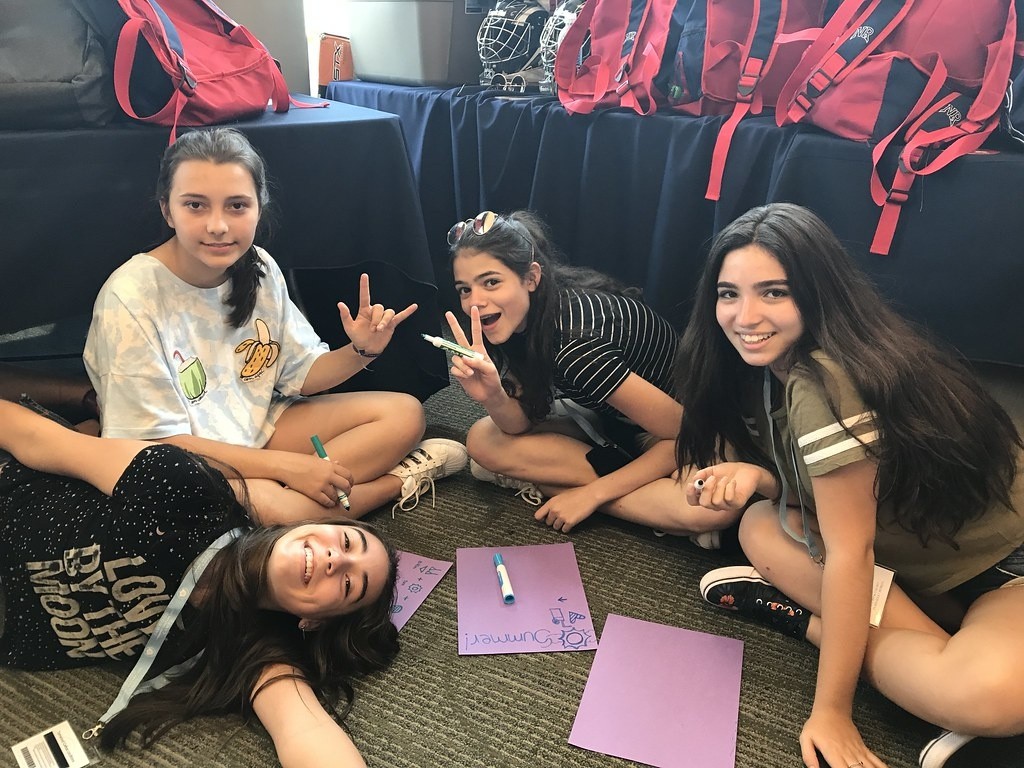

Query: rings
[849,761,863,768]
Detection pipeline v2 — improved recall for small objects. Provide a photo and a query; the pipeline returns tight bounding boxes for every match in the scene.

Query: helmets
[475,0,595,90]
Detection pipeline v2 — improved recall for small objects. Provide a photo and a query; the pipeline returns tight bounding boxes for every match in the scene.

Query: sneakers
[469,459,544,506]
[653,526,722,551]
[917,729,995,768]
[699,564,812,639]
[388,438,466,519]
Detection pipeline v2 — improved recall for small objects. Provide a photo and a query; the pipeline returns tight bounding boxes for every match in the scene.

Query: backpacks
[558,0,1017,145]
[0,0,117,128]
[72,0,288,127]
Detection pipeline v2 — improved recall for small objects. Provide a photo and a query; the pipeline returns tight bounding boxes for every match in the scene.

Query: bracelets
[352,344,382,371]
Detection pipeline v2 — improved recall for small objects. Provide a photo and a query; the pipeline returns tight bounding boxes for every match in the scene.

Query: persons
[0,398,401,768]
[84,129,469,525]
[686,203,1024,768]
[445,212,744,549]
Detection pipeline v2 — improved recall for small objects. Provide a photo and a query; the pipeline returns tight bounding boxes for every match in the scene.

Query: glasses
[446,210,533,245]
[456,74,527,95]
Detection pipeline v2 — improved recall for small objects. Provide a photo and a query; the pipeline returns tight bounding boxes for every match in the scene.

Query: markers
[421,333,484,363]
[694,479,705,497]
[492,553,515,604]
[310,435,351,511]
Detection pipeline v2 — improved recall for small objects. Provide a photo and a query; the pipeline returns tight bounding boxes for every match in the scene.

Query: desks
[0,76,1024,402]
